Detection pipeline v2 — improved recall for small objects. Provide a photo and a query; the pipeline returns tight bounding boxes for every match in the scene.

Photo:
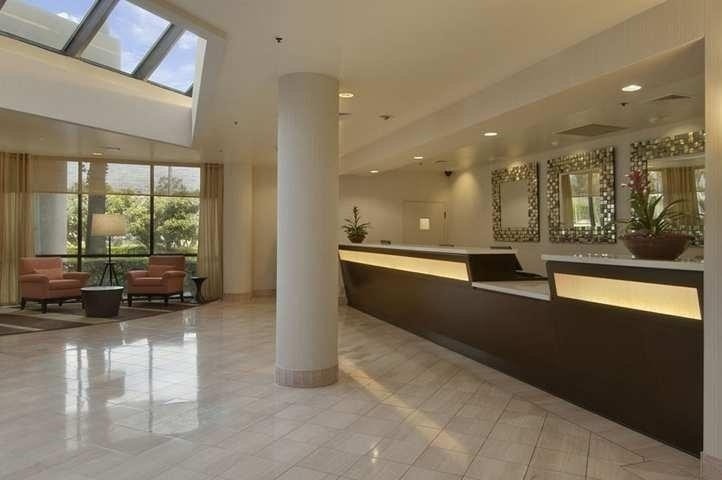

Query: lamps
[91,214,127,304]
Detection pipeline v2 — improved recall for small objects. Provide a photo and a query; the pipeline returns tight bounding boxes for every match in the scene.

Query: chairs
[19,257,90,313]
[127,255,186,306]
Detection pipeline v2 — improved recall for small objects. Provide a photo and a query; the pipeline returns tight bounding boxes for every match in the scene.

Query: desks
[191,276,208,304]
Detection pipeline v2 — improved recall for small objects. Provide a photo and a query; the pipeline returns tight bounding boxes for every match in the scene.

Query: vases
[621,233,691,260]
[347,235,365,243]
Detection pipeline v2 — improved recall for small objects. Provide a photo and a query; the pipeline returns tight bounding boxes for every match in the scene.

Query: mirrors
[490,160,540,244]
[629,130,705,248]
[546,146,617,244]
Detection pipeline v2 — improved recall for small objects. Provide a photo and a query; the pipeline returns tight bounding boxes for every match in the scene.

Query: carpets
[0,296,202,336]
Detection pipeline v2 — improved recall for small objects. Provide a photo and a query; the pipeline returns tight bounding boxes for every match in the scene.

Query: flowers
[602,160,703,235]
[341,205,370,236]
